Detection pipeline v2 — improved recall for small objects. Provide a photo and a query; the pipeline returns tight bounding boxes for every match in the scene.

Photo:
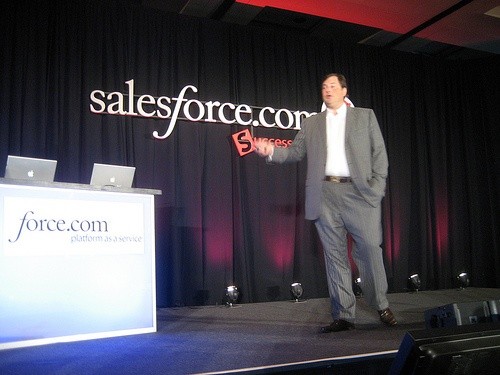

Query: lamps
[457,272,470,288]
[224,286,239,307]
[290,283,303,302]
[408,274,422,293]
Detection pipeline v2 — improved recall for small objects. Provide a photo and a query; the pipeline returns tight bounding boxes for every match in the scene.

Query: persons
[252,73,398,334]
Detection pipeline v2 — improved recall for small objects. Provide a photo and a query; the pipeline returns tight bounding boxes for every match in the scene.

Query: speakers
[424,300,500,330]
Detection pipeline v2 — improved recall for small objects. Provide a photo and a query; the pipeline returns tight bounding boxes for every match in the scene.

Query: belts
[325,176,353,184]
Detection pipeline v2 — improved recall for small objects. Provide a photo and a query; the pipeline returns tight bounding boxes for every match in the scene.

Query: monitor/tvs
[390,323,500,375]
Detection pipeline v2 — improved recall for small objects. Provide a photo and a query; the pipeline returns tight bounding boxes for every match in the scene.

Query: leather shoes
[378,307,398,326]
[320,321,355,333]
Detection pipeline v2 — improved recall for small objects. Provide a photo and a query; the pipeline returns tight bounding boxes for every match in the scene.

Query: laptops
[5,155,57,182]
[90,163,136,188]
